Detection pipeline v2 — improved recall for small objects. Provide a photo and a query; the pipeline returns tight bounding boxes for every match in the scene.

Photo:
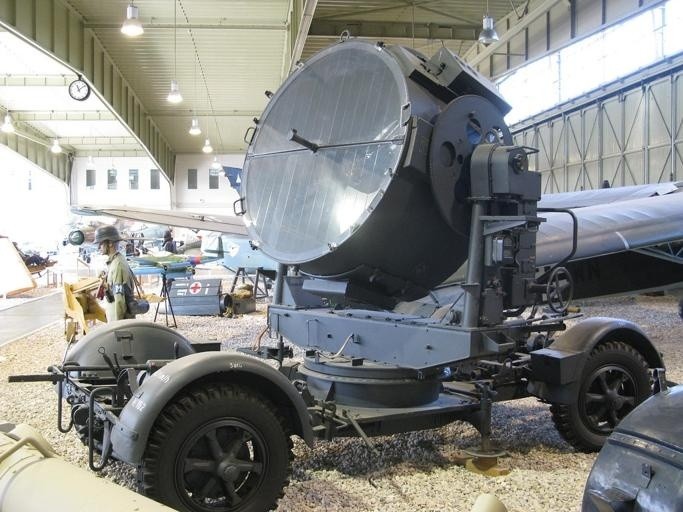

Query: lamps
[478,0,500,45]
[119,1,215,154]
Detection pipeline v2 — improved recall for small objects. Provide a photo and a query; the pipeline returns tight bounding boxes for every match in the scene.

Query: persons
[138,234,144,249]
[163,227,173,253]
[93,226,136,324]
[12,242,50,266]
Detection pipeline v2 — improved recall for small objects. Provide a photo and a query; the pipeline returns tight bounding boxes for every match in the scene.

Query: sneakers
[38,256,49,265]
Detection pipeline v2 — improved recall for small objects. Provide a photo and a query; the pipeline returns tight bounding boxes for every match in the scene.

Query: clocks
[69,80,90,101]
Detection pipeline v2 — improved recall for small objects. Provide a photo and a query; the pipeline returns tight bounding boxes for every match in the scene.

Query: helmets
[93,226,125,244]
